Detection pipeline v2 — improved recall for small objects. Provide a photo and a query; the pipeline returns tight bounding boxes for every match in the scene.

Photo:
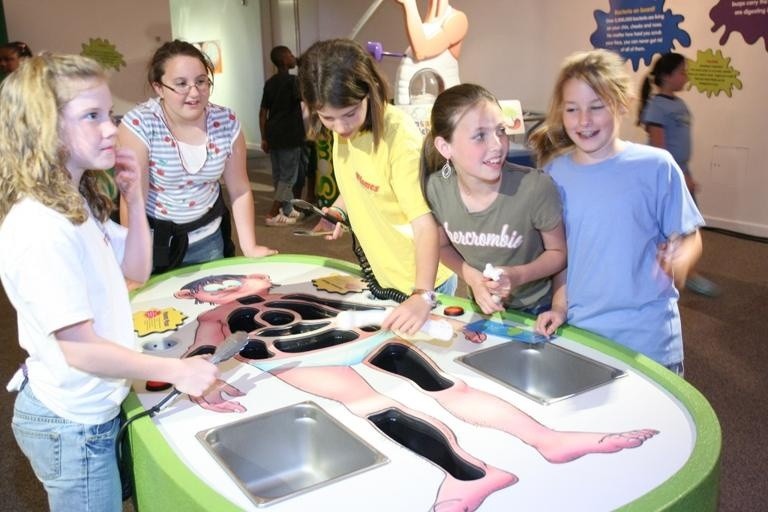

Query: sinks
[452,336,628,407]
[194,400,391,508]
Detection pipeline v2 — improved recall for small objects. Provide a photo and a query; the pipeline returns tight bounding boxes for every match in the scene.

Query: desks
[115,253,722,512]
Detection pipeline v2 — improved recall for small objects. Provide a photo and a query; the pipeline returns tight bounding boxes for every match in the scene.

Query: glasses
[158,77,214,94]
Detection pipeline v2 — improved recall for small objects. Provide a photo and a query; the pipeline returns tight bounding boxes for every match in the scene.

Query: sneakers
[264,208,296,226]
[686,275,722,297]
[289,208,300,218]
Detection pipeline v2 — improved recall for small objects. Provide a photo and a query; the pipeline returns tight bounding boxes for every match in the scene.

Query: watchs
[412,289,437,309]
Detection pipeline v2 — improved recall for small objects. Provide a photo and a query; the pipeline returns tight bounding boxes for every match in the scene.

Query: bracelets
[330,205,349,223]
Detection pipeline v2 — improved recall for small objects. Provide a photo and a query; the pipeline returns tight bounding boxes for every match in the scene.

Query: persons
[1,42,33,81]
[635,54,722,298]
[259,46,305,227]
[171,273,663,512]
[114,39,278,276]
[418,82,568,316]
[292,98,322,215]
[300,39,457,335]
[534,49,705,381]
[1,52,219,512]
